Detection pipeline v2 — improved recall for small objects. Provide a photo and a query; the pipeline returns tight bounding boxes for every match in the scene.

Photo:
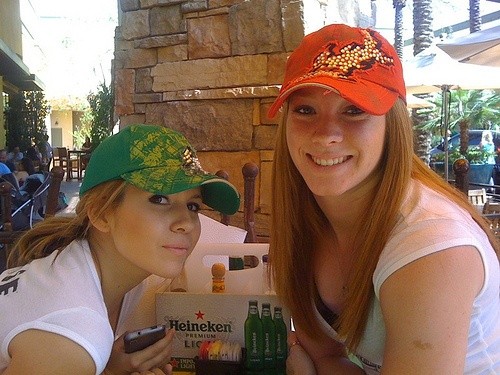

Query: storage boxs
[155,244,291,371]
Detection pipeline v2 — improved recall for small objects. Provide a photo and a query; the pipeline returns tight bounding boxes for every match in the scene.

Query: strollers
[0,162,69,230]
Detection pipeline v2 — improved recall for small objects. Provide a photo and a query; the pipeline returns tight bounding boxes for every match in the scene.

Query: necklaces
[329,235,359,293]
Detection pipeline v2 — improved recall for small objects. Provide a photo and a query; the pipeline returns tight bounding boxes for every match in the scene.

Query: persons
[492,147,500,202]
[479,130,496,196]
[0,132,53,230]
[0,123,201,375]
[81,136,93,170]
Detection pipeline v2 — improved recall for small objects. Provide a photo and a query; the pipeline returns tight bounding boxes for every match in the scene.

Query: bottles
[211,263,225,293]
[244,300,263,361]
[272,308,287,359]
[260,304,277,360]
[262,256,276,295]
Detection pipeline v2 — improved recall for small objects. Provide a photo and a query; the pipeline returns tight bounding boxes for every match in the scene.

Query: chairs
[0,181,21,275]
[482,203,500,238]
[52,147,88,182]
[45,166,65,221]
[467,188,487,205]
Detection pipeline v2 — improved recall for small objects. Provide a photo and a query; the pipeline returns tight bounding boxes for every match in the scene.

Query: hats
[79,124,241,215]
[266,24,407,121]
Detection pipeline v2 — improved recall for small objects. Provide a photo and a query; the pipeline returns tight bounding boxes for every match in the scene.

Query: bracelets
[288,341,305,352]
[266,23,500,375]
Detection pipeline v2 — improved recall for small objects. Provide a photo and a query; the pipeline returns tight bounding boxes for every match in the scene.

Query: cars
[427,130,500,195]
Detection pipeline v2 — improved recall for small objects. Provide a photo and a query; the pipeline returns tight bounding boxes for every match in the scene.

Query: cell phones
[124,324,166,353]
